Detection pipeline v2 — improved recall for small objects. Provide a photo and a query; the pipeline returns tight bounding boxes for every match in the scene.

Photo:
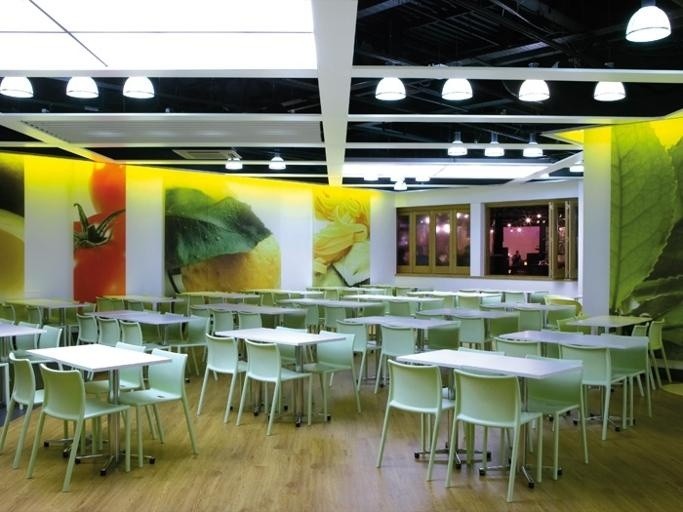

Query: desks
[202,301,308,322]
[181,290,261,302]
[9,297,84,322]
[214,326,345,429]
[85,309,189,330]
[101,289,174,309]
[0,319,44,348]
[347,293,443,302]
[495,330,647,431]
[26,346,174,477]
[282,296,383,315]
[344,312,456,332]
[563,313,649,330]
[395,350,581,488]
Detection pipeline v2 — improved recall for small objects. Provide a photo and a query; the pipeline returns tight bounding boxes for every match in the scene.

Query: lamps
[373,77,407,101]
[65,77,99,100]
[625,0,671,45]
[123,77,154,99]
[225,158,243,169]
[593,81,627,103]
[523,132,542,158]
[0,77,35,99]
[443,78,472,103]
[519,78,549,103]
[447,132,468,155]
[268,155,286,169]
[485,131,505,158]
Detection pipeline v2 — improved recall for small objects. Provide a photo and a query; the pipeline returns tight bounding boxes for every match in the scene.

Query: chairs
[457,316,491,350]
[37,325,62,350]
[196,334,250,422]
[649,318,673,388]
[114,350,198,469]
[0,353,67,470]
[377,360,457,480]
[517,308,542,330]
[237,313,262,330]
[602,336,652,427]
[161,313,184,381]
[82,343,156,446]
[14,321,37,352]
[329,320,386,391]
[445,367,544,500]
[119,320,167,351]
[458,347,503,356]
[303,331,361,422]
[557,319,576,333]
[373,326,419,393]
[490,335,541,359]
[558,345,627,441]
[96,315,119,346]
[631,321,656,401]
[210,311,235,331]
[172,317,204,374]
[236,334,312,435]
[74,313,98,345]
[273,326,305,366]
[26,362,130,493]
[522,354,589,480]
[308,284,550,325]
